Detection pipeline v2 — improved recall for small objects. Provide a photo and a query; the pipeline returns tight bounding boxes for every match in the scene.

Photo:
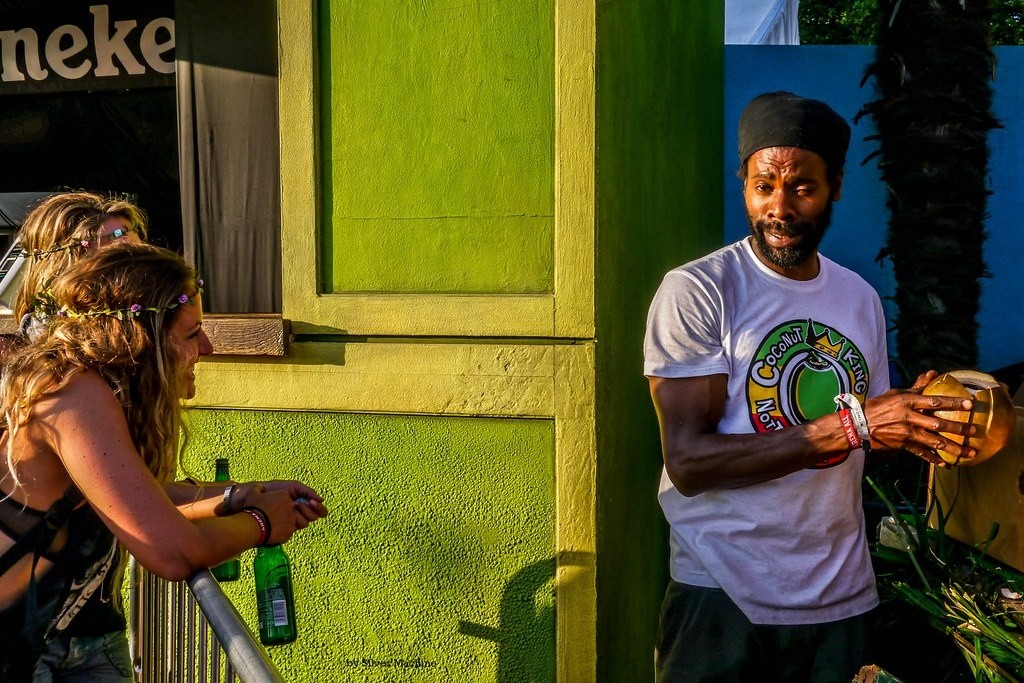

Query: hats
[738,91,851,168]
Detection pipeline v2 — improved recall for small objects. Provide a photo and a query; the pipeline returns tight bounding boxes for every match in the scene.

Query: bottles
[252,543,298,647]
[210,458,243,581]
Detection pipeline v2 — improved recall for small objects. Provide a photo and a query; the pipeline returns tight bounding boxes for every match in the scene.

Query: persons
[643,90,978,683]
[0,193,148,683]
[0,243,328,683]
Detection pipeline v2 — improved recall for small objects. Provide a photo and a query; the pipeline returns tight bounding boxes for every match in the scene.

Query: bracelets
[240,506,271,548]
[223,484,234,515]
[834,393,869,439]
[838,409,862,448]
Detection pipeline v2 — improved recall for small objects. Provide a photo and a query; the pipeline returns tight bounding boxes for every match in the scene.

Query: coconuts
[919,370,1014,467]
